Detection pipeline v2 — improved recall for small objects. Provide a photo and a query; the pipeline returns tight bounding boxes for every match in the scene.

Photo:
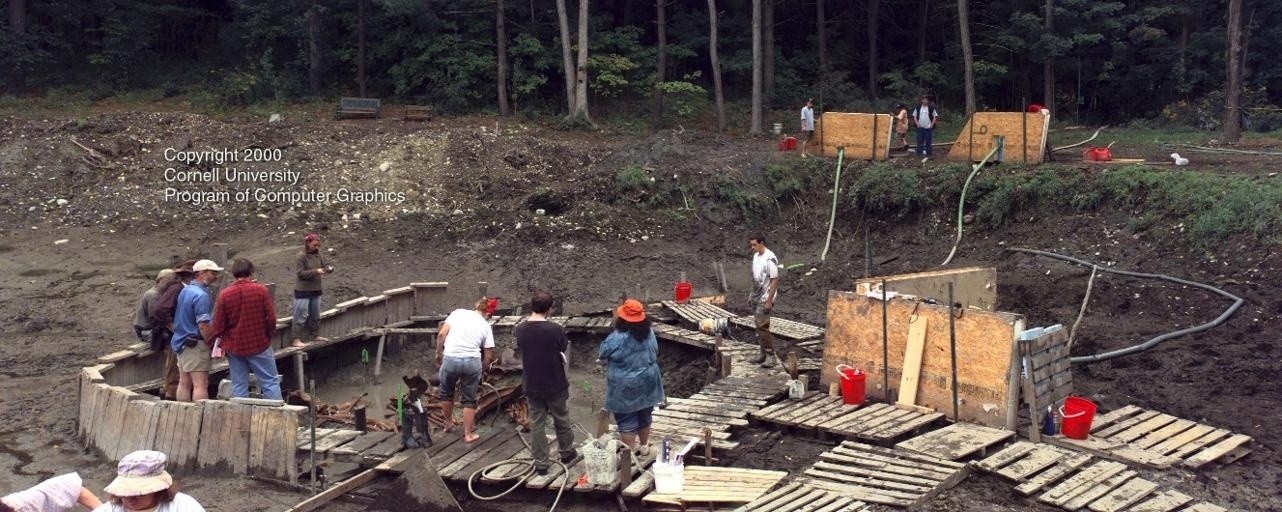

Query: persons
[212,258,282,400]
[290,234,328,347]
[434,297,498,443]
[800,98,816,158]
[599,299,665,466]
[891,103,909,149]
[747,232,779,367]
[134,268,176,342]
[0,471,103,512]
[912,95,939,157]
[152,260,195,399]
[171,259,224,401]
[92,450,206,512]
[516,291,577,475]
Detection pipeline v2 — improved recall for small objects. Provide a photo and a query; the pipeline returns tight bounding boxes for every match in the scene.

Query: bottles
[1045,405,1054,437]
[1052,413,1060,434]
[828,377,840,396]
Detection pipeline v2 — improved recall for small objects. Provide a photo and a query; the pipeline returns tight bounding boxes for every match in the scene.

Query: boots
[400,408,433,449]
[750,348,778,368]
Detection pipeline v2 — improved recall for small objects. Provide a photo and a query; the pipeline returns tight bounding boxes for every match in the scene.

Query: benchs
[403,104,433,121]
[336,97,381,121]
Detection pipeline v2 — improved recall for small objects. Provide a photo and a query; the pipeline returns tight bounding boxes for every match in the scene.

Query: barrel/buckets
[700,316,734,338]
[1096,147,1111,162]
[580,438,618,485]
[835,363,866,404]
[1058,395,1098,440]
[652,460,684,494]
[1086,146,1096,161]
[787,136,796,150]
[772,122,783,134]
[673,279,691,304]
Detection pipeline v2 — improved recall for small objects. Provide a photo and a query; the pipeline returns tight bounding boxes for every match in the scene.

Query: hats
[617,299,645,322]
[156,258,225,280]
[105,450,172,498]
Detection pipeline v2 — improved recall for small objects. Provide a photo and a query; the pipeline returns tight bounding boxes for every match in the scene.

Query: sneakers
[537,469,548,475]
[561,448,576,464]
[637,443,650,455]
[801,151,815,159]
